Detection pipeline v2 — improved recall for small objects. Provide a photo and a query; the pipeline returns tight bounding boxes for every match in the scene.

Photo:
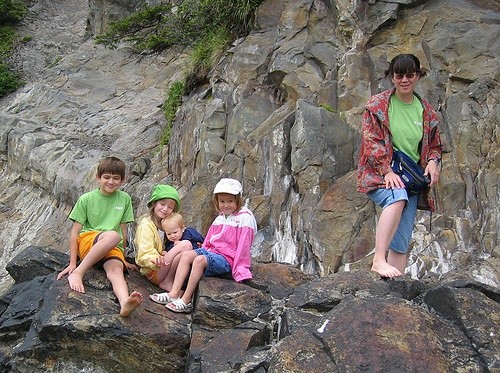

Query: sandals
[149,293,180,305]
[164,299,193,313]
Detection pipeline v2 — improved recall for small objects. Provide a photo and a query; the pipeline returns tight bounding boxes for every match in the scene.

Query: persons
[149,177,258,314]
[133,184,205,298]
[356,53,443,278]
[56,157,144,318]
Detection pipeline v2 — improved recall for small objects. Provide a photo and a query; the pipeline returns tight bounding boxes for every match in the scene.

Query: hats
[213,178,243,197]
[146,185,181,213]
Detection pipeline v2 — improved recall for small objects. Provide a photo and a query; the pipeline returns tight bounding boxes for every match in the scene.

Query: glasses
[393,74,417,79]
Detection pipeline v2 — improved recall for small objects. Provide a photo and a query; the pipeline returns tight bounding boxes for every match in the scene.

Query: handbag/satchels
[390,150,431,195]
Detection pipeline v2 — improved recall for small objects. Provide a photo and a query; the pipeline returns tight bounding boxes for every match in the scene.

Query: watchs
[427,158,439,166]
